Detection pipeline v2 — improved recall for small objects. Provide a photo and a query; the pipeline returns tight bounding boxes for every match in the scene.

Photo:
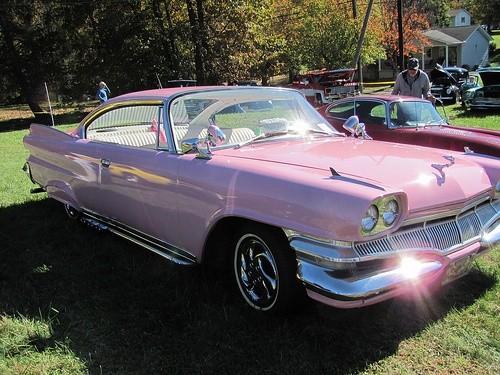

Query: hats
[408,58,419,68]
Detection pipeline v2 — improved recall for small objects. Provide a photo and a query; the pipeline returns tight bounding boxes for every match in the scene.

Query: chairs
[357,103,373,124]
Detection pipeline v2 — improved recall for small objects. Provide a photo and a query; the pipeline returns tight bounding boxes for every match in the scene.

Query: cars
[464,68,500,108]
[429,68,479,104]
[317,97,500,158]
[22,89,500,315]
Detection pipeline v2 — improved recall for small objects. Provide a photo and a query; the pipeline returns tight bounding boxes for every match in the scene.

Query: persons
[97,81,111,105]
[391,58,435,113]
[232,81,245,113]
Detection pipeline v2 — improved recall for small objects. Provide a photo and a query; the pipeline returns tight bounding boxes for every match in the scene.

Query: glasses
[409,69,416,70]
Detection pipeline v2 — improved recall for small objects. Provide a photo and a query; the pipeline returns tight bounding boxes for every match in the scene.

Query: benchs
[91,127,256,154]
